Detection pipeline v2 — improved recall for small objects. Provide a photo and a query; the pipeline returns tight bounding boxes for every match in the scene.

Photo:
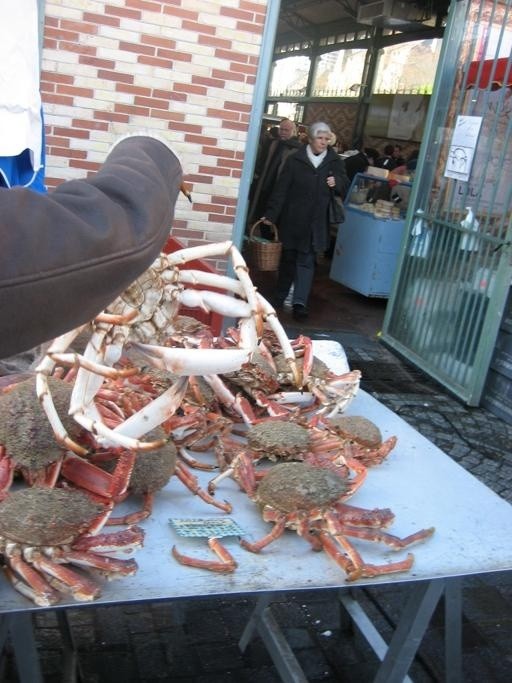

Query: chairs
[292,304,310,320]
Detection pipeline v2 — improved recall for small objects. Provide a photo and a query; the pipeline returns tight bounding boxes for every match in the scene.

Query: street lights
[329,198,345,224]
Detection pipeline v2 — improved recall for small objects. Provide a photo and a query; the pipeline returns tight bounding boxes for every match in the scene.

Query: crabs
[37,241,304,456]
[0,317,438,603]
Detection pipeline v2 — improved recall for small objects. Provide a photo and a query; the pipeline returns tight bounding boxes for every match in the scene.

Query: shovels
[375,200,399,218]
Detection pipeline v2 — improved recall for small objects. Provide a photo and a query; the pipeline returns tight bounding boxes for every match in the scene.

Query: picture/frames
[0,360,511,683]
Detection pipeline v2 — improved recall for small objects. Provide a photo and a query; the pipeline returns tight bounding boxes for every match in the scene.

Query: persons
[0,131,184,359]
[246,119,419,322]
